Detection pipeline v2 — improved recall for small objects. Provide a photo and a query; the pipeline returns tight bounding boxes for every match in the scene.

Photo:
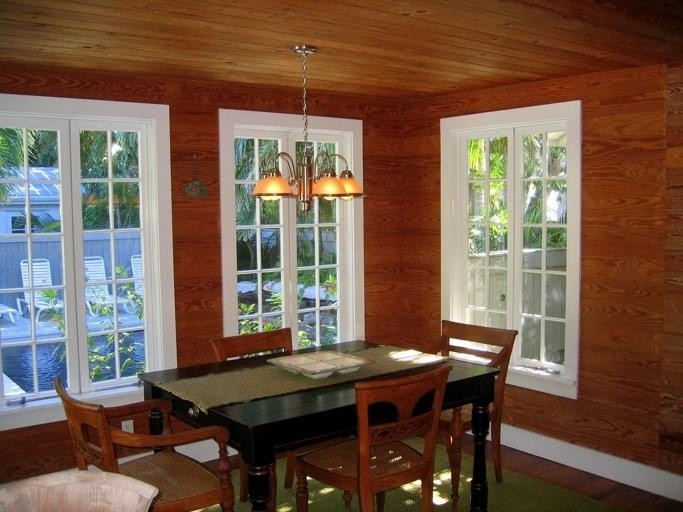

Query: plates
[265,350,377,380]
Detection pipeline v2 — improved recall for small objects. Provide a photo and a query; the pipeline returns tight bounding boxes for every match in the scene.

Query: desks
[137,341,500,509]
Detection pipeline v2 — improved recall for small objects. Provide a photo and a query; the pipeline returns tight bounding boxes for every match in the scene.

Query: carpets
[196,429,632,511]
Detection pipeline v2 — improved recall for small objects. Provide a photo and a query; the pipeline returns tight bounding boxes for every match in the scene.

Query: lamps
[250,45,367,209]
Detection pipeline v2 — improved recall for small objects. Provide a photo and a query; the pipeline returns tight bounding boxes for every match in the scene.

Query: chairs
[215,327,295,502]
[294,364,452,509]
[0,255,144,325]
[55,376,236,510]
[404,321,518,509]
